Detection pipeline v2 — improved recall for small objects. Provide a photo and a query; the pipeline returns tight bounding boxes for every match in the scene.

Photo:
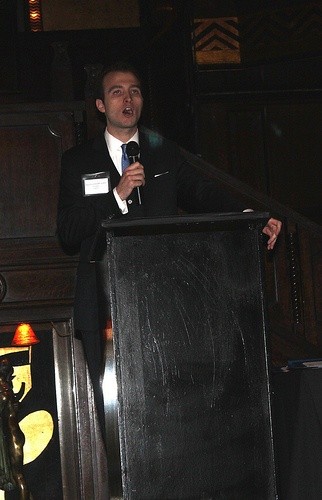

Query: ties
[121,144,130,172]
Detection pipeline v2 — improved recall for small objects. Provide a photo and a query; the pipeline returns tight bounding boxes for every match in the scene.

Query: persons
[57,64,282,456]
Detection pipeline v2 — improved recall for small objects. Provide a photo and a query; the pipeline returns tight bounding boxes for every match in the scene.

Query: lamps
[12,323,41,346]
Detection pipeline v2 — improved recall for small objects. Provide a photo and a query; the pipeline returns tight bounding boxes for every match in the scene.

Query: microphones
[126,141,142,206]
